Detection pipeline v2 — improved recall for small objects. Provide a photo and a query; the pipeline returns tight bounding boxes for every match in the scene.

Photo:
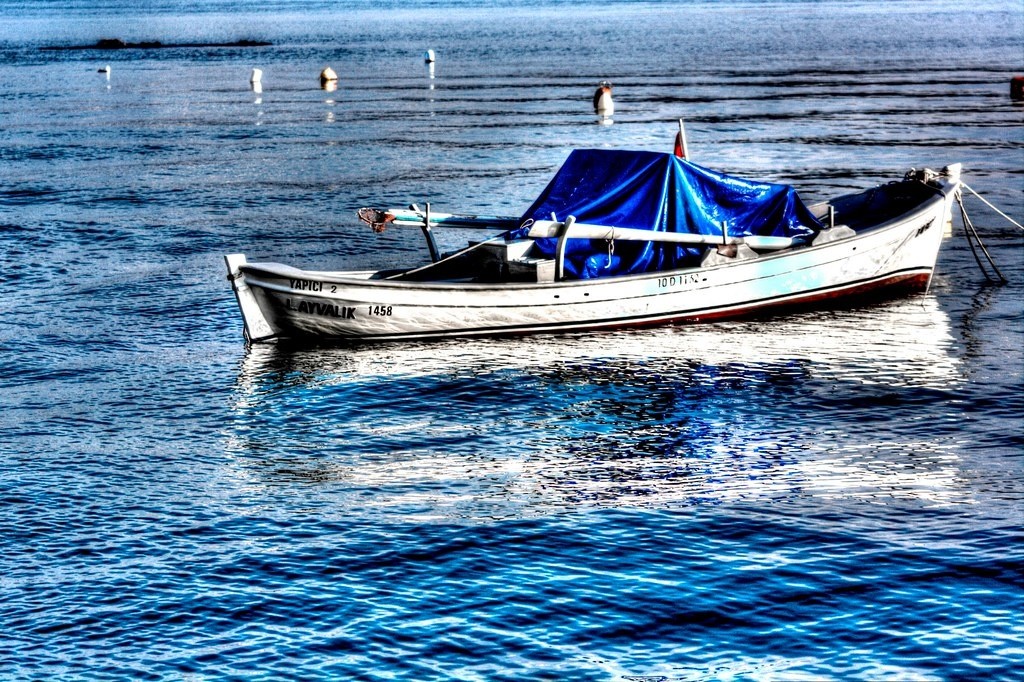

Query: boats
[223,116,962,346]
[198,309,973,519]
